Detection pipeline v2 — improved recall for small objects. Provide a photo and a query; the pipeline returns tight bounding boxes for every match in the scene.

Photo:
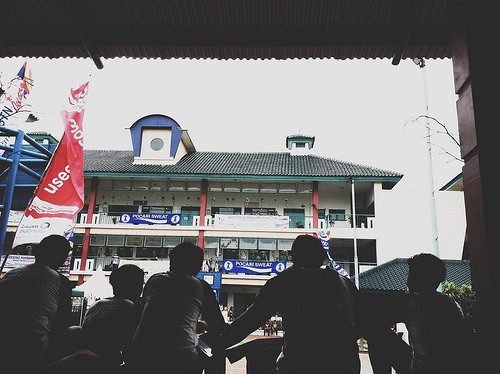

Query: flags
[11,79,90,249]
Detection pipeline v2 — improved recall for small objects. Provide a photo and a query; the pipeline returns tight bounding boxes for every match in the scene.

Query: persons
[0,235,84,374]
[225,236,371,374]
[81,265,144,374]
[369,253,477,374]
[123,242,225,374]
